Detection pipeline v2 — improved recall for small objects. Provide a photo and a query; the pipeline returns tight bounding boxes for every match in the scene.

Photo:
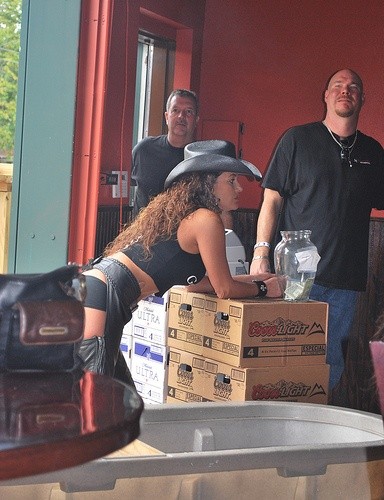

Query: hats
[164,139,262,192]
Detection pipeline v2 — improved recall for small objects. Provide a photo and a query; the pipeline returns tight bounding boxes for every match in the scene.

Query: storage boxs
[120,286,330,404]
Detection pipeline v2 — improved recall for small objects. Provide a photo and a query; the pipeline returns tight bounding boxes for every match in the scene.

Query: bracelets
[251,256,270,261]
[254,279,267,297]
[251,241,270,249]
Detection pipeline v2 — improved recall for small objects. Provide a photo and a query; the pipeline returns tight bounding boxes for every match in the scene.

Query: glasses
[339,135,352,168]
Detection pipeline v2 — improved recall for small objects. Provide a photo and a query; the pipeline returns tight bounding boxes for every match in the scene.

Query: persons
[129,90,201,225]
[76,138,288,375]
[250,68,384,396]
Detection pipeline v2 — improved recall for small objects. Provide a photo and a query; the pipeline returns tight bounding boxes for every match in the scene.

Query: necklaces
[322,121,359,158]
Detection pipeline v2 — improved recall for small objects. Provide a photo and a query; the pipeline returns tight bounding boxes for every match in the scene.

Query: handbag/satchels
[0,263,87,372]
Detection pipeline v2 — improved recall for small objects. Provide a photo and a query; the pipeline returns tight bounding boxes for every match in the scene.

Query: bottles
[273,229,318,304]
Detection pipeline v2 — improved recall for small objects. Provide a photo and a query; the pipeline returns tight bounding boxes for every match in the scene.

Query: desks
[0,371,143,479]
